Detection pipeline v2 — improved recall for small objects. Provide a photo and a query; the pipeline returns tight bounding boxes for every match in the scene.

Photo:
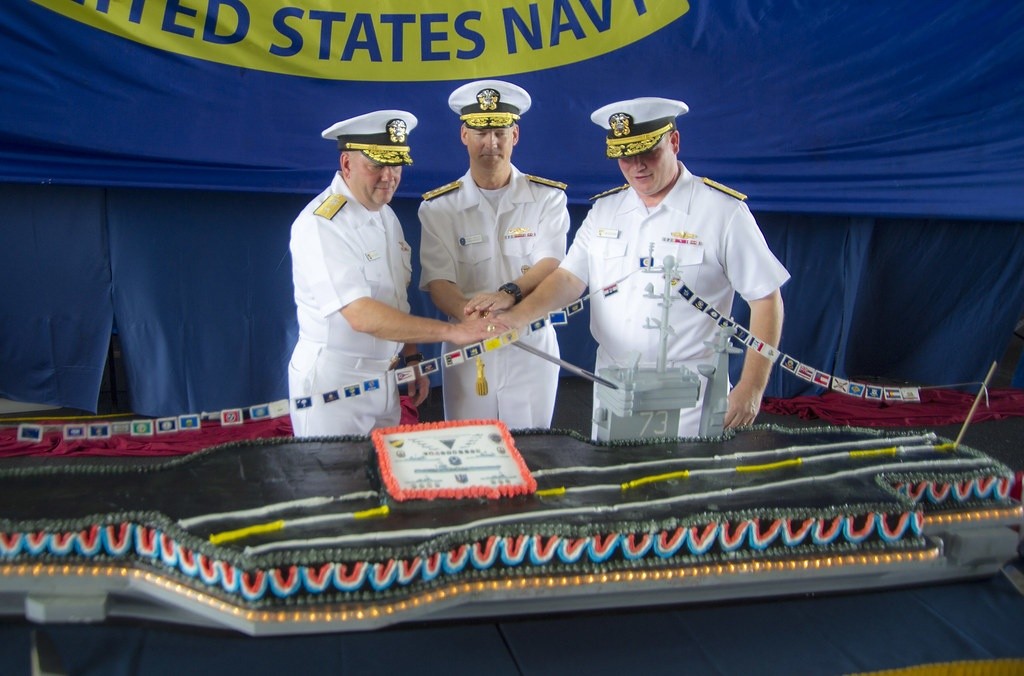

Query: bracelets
[481,311,489,318]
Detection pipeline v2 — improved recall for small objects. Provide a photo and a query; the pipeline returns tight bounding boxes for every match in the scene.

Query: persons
[287,80,791,438]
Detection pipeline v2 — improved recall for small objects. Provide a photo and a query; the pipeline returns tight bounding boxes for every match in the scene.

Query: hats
[448,79,531,129]
[590,97,689,159]
[321,108,418,167]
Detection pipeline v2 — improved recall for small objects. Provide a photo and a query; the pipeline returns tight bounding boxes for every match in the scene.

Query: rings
[486,324,495,333]
[740,422,746,426]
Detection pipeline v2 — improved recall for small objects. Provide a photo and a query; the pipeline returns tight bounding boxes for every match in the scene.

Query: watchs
[498,282,522,306]
[403,352,424,365]
